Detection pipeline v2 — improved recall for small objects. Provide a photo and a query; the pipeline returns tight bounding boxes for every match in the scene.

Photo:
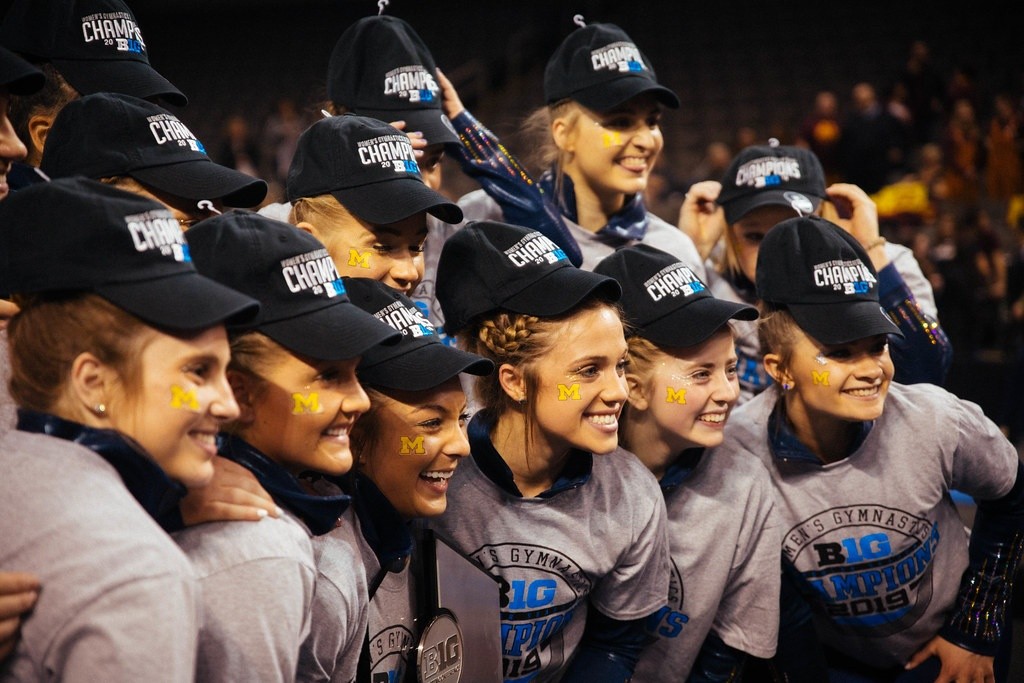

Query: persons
[436,221,671,683]
[723,217,1024,682]
[0,1,1024,682]
[458,23,706,285]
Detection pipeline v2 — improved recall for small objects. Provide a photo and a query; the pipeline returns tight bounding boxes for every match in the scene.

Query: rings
[685,193,689,198]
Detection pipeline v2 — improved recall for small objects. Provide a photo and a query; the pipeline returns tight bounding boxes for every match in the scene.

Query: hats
[0,0,188,117]
[591,243,760,350]
[325,15,463,159]
[181,208,404,361]
[0,178,260,344]
[714,140,825,225]
[541,23,681,118]
[38,93,268,209]
[433,220,622,340]
[0,51,46,96]
[342,275,495,395]
[755,217,907,347]
[287,113,463,226]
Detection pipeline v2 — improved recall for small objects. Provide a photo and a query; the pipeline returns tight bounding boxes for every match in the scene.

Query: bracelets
[867,238,886,249]
